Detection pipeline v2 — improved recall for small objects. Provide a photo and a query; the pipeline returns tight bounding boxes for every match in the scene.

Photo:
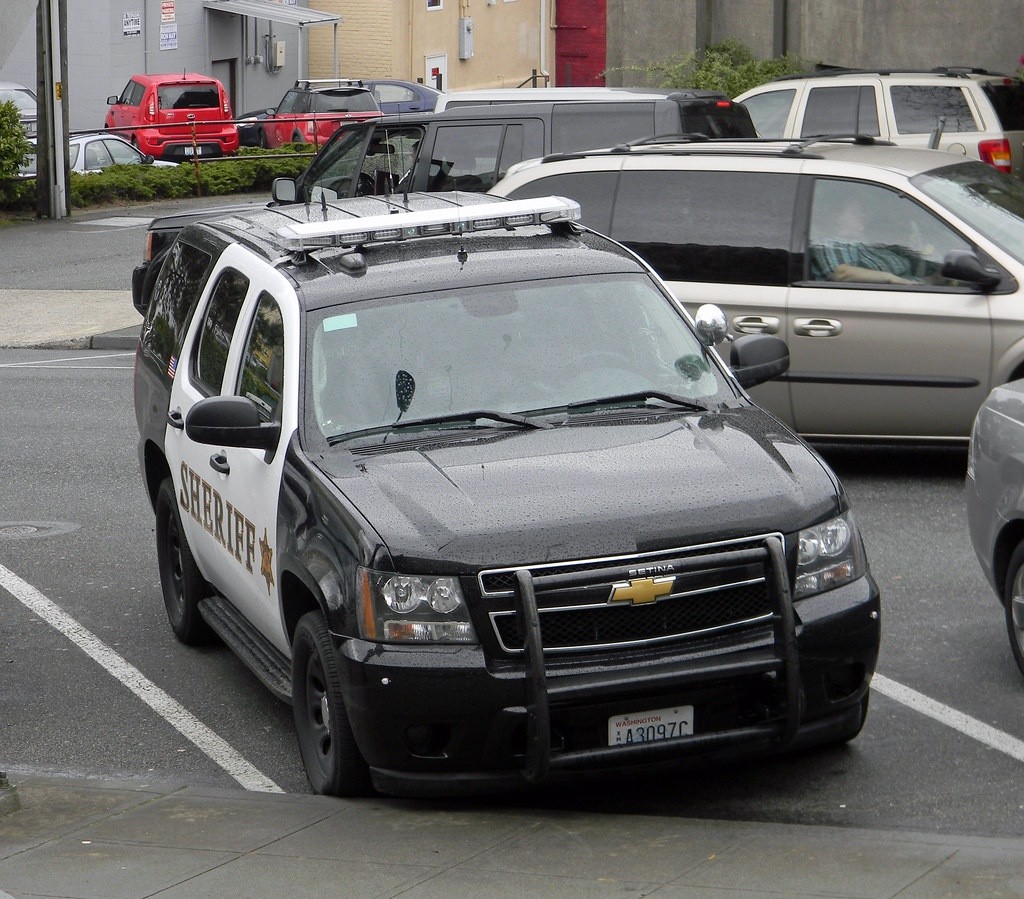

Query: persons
[812,191,944,285]
[393,140,420,193]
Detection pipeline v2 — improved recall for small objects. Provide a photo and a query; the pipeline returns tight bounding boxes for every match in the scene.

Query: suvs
[256,77,388,151]
[130,187,884,801]
[732,61,1024,180]
[472,130,1024,463]
[266,99,760,210]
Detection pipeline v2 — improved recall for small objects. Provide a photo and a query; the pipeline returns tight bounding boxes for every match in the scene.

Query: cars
[104,72,241,162]
[15,131,181,179]
[958,373,1024,678]
[234,110,274,147]
[0,81,39,139]
[347,78,445,112]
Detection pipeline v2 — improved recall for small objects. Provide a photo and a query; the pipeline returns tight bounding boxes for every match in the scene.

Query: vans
[430,86,731,167]
